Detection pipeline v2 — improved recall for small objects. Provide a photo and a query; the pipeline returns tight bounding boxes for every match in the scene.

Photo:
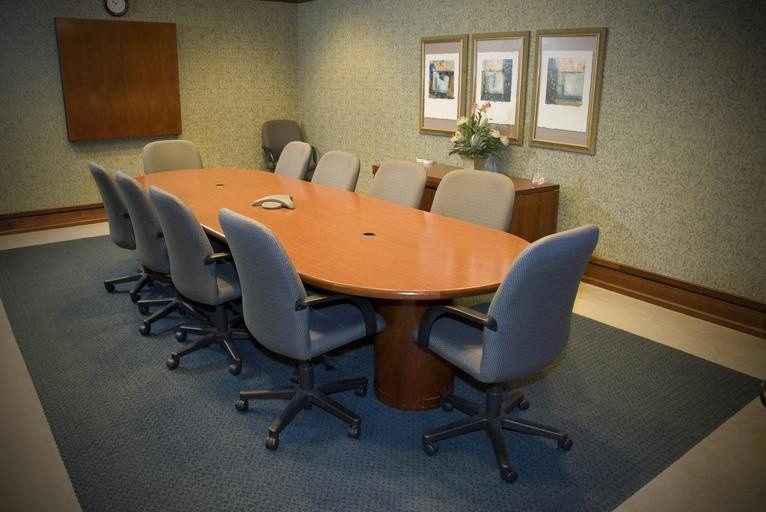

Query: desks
[372,161,560,244]
[128,168,534,412]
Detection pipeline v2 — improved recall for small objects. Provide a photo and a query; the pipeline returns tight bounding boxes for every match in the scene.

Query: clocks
[103,0,129,17]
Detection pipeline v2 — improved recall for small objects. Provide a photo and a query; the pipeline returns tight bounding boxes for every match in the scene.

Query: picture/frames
[524,25,612,158]
[464,28,532,148]
[417,31,469,137]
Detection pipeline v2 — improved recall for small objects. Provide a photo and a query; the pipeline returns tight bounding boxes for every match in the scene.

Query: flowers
[444,99,514,160]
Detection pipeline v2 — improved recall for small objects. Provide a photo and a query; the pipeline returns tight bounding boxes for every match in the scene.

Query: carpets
[0,225,765,511]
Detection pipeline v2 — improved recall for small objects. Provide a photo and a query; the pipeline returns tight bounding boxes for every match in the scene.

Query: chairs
[115,170,216,336]
[142,140,204,174]
[367,158,427,208]
[411,226,600,484]
[262,119,317,180]
[430,169,515,232]
[218,207,388,451]
[147,185,252,376]
[88,162,172,304]
[312,150,360,193]
